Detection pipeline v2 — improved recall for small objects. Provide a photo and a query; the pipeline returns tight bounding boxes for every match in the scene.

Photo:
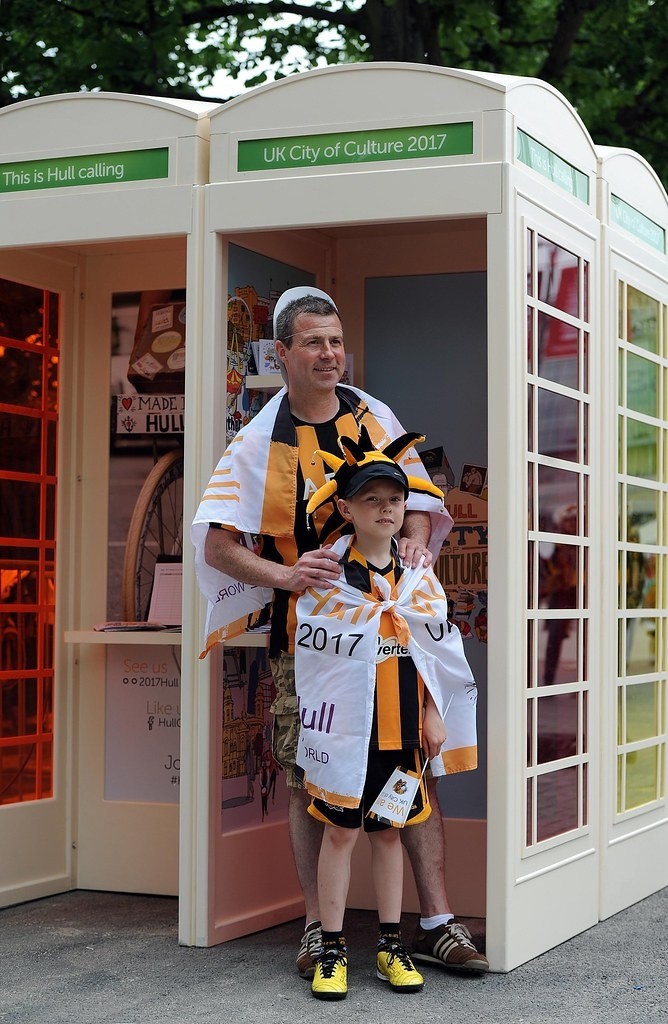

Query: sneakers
[410,914,490,970]
[296,921,323,977]
[376,942,424,991]
[311,945,348,997]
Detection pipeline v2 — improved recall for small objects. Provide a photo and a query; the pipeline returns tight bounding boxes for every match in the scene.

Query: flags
[367,766,420,828]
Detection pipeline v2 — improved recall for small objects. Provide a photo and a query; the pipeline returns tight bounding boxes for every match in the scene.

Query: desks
[62,625,271,647]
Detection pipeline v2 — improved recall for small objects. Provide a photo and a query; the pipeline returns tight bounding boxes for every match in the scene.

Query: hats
[305,423,445,545]
[273,286,338,388]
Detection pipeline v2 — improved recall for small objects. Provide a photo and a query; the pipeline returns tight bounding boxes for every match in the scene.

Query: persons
[204,286,489,977]
[540,502,579,685]
[287,454,477,1001]
